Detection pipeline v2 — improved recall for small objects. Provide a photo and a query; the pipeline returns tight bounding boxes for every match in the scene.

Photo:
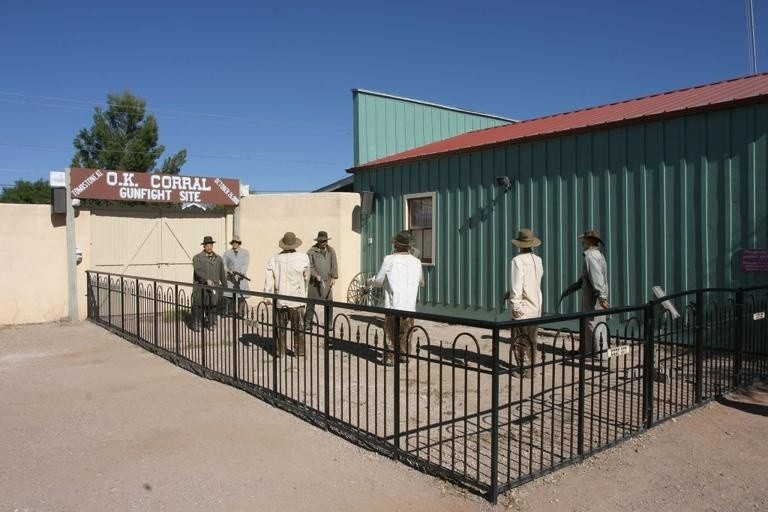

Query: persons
[222,235,249,318]
[365,230,422,367]
[193,236,228,331]
[563,230,609,361]
[503,229,544,379]
[263,232,311,359]
[305,231,338,331]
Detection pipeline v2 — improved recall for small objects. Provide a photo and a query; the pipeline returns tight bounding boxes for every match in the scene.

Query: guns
[557,289,569,307]
[227,271,251,282]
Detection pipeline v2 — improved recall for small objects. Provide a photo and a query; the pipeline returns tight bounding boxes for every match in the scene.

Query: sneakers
[377,353,394,366]
[324,323,333,331]
[304,324,312,330]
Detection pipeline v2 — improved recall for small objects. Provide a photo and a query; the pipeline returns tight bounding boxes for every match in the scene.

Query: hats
[201,237,215,245]
[578,230,602,242]
[510,229,541,249]
[279,232,302,250]
[230,236,241,245]
[314,232,331,241]
[392,231,415,246]
